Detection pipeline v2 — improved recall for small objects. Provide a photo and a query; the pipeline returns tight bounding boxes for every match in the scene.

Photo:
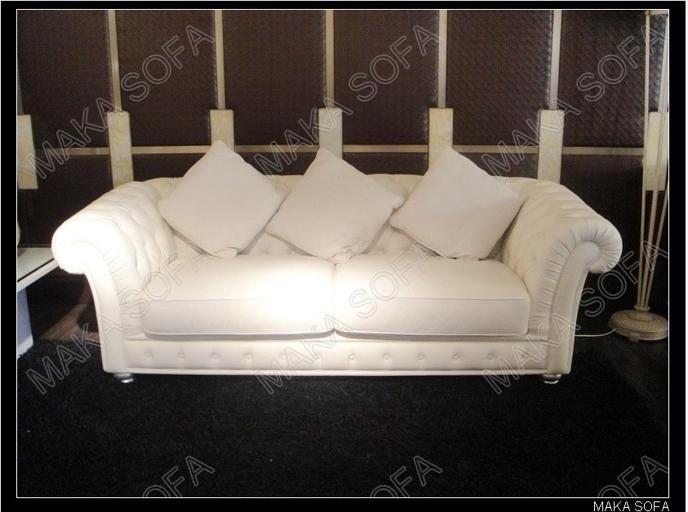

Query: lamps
[610,11,668,342]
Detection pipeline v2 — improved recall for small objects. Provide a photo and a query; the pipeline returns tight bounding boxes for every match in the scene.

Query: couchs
[51,164,622,385]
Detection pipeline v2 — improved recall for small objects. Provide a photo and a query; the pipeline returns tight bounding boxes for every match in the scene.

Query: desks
[15,245,58,362]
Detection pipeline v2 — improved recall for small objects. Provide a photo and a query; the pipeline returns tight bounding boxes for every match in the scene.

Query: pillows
[267,147,405,261]
[390,145,529,262]
[161,142,286,258]
